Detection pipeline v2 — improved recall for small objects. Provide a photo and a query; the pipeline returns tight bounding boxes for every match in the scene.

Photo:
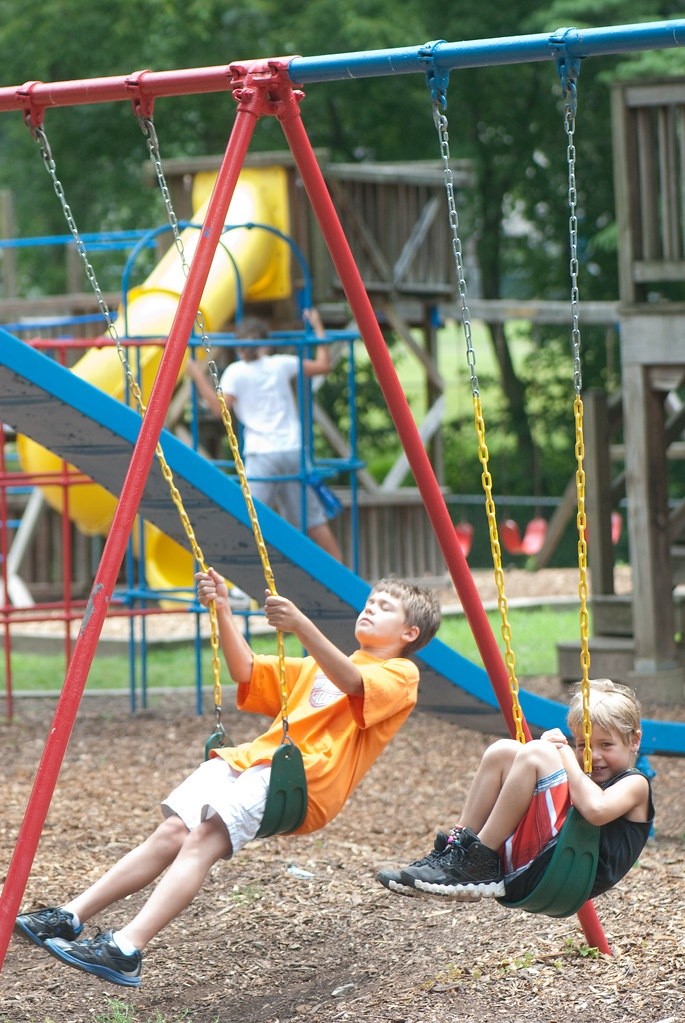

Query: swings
[416,25,613,922]
[16,69,309,846]
[409,319,625,564]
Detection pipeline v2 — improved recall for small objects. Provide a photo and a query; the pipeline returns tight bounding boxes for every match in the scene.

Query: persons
[12,567,442,987]
[378,679,656,904]
[184,308,344,567]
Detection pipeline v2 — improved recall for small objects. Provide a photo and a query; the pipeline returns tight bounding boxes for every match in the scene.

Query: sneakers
[401,824,506,899]
[13,904,84,947]
[43,926,144,987]
[376,831,482,902]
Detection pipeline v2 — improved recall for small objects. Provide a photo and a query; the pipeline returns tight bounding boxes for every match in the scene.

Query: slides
[18,176,273,594]
